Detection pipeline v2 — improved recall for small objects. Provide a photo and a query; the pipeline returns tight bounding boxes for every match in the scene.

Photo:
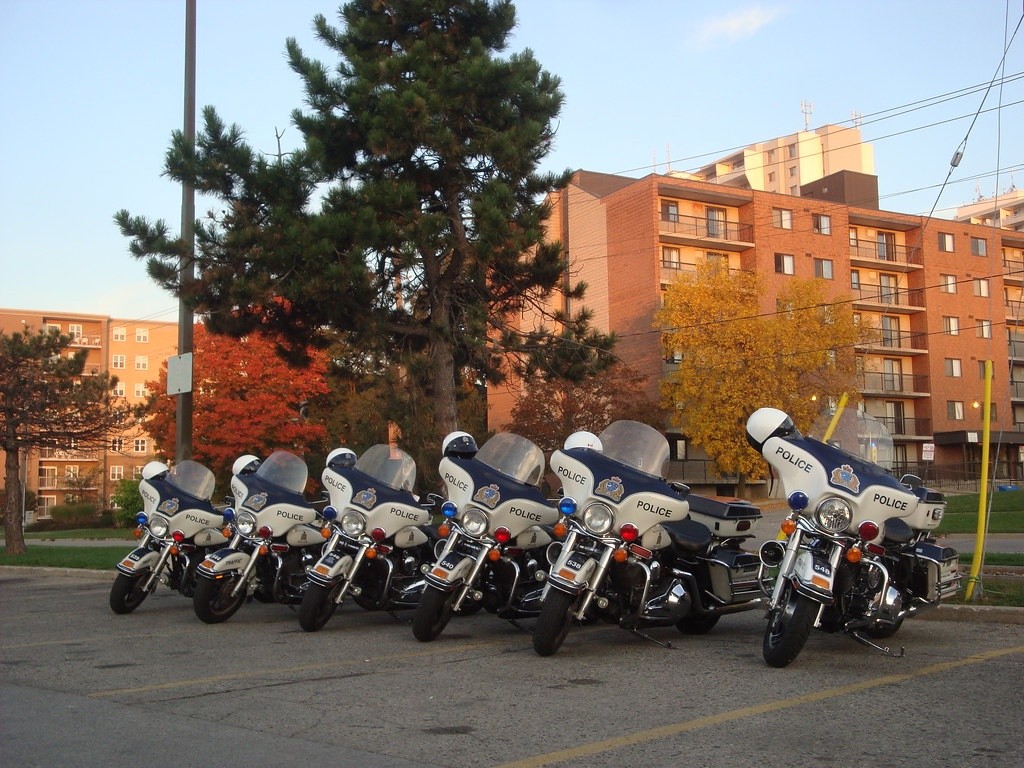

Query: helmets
[140,461,170,480]
[324,448,361,470]
[743,406,795,453]
[562,430,602,455]
[441,432,479,461]
[231,455,262,478]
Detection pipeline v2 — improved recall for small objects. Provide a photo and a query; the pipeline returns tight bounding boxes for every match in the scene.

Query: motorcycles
[298,442,486,639]
[191,448,392,628]
[108,458,285,613]
[745,403,962,671]
[532,418,773,657]
[412,424,605,648]
